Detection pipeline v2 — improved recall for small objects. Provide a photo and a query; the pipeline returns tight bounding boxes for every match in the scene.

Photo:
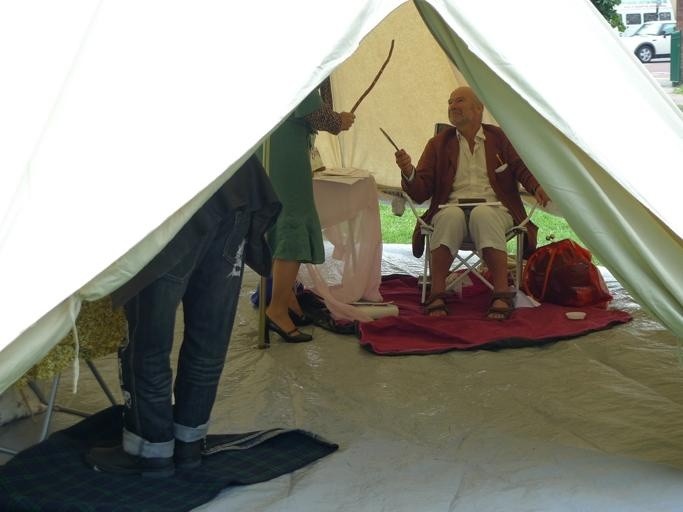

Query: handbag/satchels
[521,238,614,308]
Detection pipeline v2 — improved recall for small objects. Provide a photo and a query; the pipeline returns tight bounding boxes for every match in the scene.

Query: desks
[301,168,372,304]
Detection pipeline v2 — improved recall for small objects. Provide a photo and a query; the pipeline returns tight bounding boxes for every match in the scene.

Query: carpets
[303,270,639,355]
[0,402,340,510]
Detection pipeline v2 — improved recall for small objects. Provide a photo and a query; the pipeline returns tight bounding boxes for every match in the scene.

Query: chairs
[385,186,545,307]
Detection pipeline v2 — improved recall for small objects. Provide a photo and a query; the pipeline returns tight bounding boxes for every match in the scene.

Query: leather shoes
[84,444,176,480]
[173,438,205,465]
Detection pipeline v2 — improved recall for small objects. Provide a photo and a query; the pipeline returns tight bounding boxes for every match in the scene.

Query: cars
[619,21,677,64]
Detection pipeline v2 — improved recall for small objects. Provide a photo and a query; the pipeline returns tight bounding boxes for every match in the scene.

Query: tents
[0,0,682,510]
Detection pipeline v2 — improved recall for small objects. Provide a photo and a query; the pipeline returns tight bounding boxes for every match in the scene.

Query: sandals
[426,291,450,318]
[485,290,518,321]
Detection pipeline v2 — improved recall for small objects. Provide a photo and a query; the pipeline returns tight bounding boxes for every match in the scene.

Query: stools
[0,328,115,466]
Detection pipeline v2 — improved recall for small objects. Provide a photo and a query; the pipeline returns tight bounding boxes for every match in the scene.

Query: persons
[255,87,355,345]
[81,152,284,479]
[393,85,551,323]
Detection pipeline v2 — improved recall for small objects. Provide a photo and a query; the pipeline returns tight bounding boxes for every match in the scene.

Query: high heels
[288,306,313,327]
[264,312,313,343]
